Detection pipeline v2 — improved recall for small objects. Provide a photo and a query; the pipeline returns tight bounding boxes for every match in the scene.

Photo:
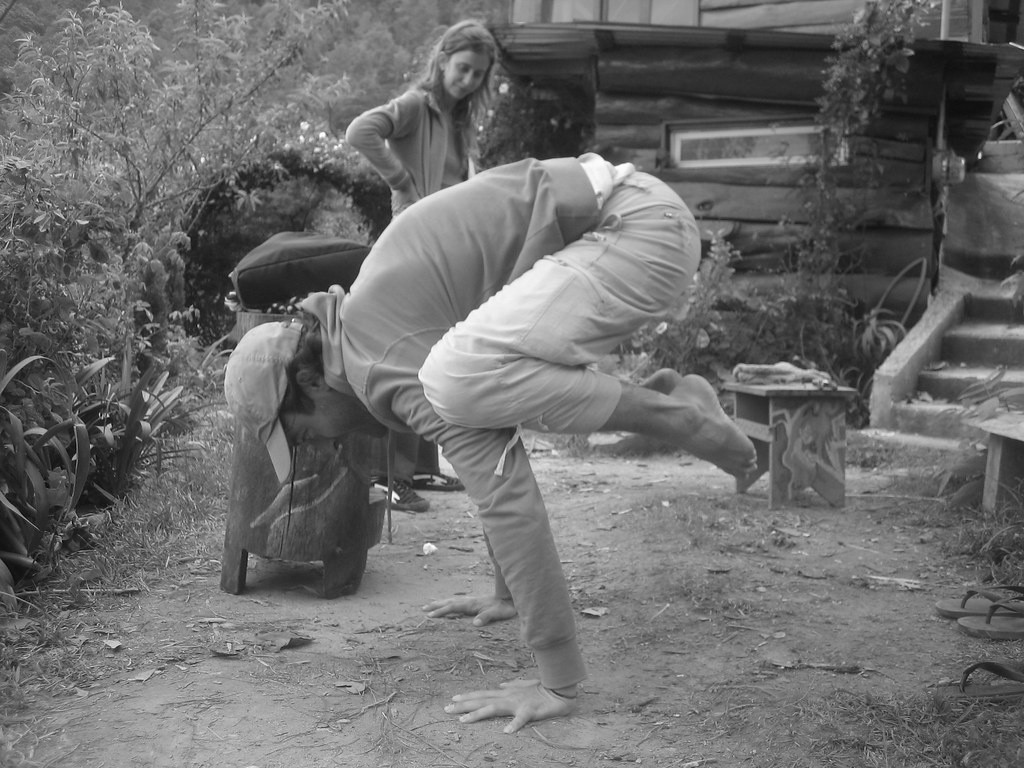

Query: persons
[345,18,497,513]
[224,152,759,734]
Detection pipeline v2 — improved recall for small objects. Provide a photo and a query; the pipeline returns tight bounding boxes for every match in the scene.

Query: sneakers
[373,477,430,513]
[411,473,465,492]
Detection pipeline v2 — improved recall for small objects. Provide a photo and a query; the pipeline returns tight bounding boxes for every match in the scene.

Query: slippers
[938,661,1024,706]
[933,585,1024,641]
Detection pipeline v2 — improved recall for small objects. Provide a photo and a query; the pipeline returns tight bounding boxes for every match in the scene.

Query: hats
[222,319,305,483]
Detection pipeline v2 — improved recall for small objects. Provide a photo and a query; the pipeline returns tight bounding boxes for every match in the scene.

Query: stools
[725,361,858,512]
[962,408,1024,525]
